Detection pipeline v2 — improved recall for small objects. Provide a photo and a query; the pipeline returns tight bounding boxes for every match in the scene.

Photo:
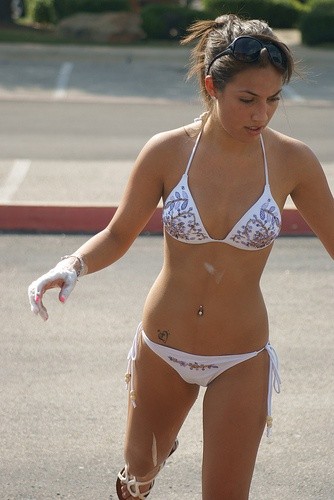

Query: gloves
[27,255,85,321]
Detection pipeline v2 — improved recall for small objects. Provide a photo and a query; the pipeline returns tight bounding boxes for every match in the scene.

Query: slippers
[116,437,180,500]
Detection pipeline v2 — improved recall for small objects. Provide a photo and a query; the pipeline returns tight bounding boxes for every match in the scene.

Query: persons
[27,18,334,499]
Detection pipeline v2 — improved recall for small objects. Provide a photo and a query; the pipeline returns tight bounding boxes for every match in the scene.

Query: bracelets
[60,256,84,275]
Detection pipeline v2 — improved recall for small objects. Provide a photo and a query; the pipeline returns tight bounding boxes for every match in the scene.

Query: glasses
[206,35,288,76]
